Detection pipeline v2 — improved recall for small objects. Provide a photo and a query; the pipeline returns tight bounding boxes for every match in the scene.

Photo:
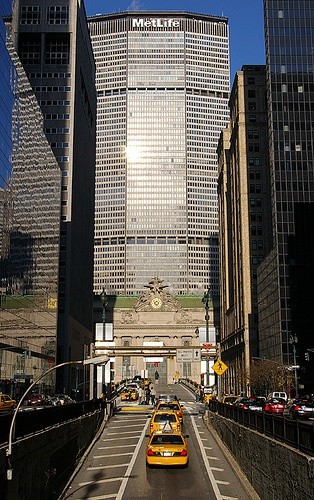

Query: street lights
[201,286,212,404]
[6,355,111,480]
[99,287,109,399]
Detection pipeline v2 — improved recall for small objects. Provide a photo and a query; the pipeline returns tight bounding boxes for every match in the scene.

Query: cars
[121,378,152,401]
[144,430,191,466]
[0,392,16,410]
[155,393,182,401]
[221,392,314,421]
[153,401,184,424]
[27,392,76,411]
[147,411,182,434]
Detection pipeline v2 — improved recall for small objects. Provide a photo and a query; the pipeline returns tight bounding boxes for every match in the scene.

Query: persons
[137,386,156,405]
[154,371,159,385]
[103,382,106,394]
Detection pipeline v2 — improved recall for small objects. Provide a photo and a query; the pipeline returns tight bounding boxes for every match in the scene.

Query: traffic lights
[305,353,309,362]
[252,360,256,366]
